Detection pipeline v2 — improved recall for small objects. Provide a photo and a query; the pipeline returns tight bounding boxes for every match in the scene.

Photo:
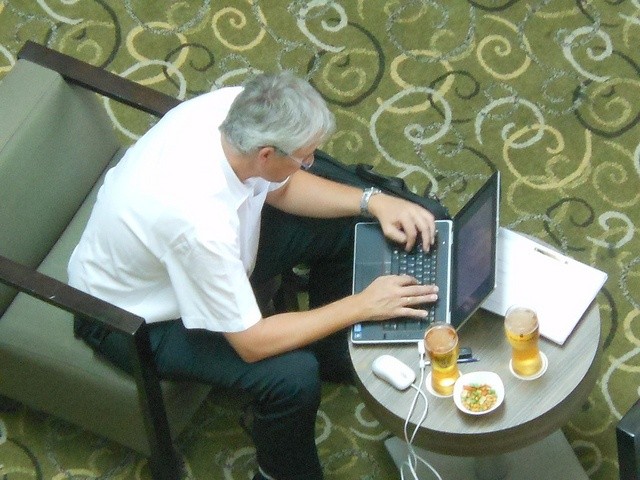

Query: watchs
[360,187,381,217]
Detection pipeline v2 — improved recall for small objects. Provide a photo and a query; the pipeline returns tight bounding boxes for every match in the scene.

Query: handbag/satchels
[315,150,449,219]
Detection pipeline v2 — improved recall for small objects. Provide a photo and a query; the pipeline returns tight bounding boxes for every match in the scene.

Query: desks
[345,225,602,480]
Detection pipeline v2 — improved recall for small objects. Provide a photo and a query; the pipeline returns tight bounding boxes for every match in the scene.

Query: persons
[68,66,440,480]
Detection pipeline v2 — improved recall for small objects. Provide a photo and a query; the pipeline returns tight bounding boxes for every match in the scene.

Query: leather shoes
[319,351,358,385]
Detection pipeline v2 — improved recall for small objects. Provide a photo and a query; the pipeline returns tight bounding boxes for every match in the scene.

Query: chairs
[615,396,639,479]
[0,38,299,479]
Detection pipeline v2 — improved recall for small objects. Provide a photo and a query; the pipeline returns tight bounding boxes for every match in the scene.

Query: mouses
[371,354,418,390]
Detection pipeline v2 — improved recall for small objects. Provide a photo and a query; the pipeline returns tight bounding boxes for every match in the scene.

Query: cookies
[460,383,497,412]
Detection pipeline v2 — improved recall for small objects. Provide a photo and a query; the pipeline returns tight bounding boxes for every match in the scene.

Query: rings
[407,297,410,305]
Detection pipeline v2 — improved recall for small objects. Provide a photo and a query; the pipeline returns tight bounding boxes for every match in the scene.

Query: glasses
[286,152,315,170]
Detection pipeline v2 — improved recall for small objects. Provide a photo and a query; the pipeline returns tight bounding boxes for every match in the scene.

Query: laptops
[350,169,502,346]
[478,224,609,347]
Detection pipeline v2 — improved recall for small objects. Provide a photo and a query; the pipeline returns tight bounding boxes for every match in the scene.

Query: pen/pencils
[424,359,481,366]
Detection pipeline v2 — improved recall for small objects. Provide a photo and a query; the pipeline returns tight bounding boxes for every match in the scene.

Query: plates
[509,351,548,380]
[425,371,462,398]
[454,370,504,416]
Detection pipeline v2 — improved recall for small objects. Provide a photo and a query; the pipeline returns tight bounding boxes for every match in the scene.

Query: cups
[424,326,460,396]
[505,305,542,377]
